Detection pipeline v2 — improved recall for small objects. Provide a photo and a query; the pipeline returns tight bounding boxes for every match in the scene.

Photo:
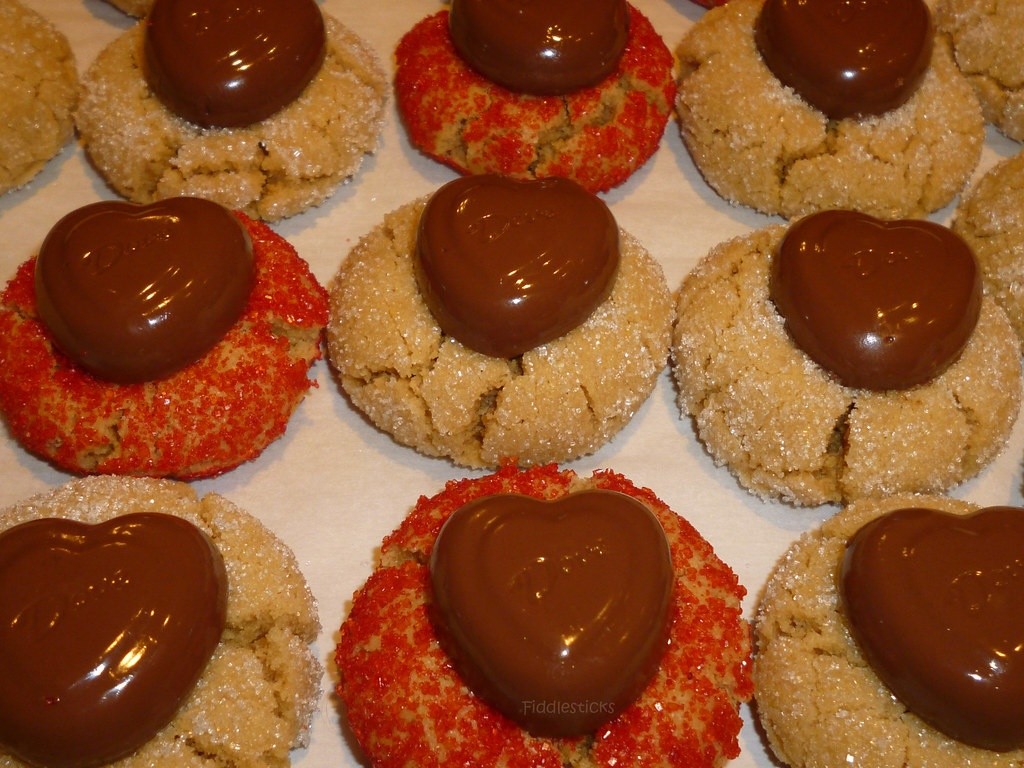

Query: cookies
[2,0,1024,768]
